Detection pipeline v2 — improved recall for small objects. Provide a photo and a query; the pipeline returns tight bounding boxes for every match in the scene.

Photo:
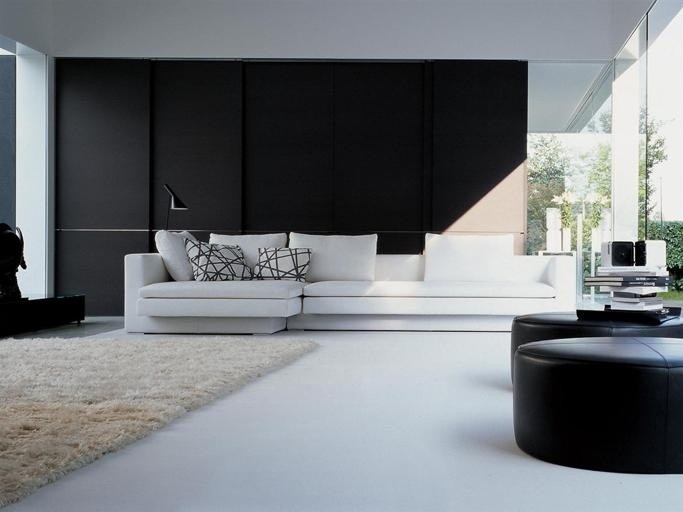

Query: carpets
[0,335,321,512]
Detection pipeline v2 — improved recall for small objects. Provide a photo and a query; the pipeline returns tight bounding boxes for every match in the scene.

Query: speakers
[601,240,634,266]
[635,240,667,266]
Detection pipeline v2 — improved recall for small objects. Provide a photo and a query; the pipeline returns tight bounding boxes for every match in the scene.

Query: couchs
[125,249,577,339]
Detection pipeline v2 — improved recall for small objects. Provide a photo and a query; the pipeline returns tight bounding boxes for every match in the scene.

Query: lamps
[160,184,187,232]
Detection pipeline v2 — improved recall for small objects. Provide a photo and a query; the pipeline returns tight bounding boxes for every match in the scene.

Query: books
[585,267,669,310]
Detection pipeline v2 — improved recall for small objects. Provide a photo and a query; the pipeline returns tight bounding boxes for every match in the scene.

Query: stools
[507,301,681,477]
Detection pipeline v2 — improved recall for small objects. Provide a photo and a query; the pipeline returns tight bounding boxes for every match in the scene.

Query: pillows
[152,222,378,280]
[423,231,516,288]
